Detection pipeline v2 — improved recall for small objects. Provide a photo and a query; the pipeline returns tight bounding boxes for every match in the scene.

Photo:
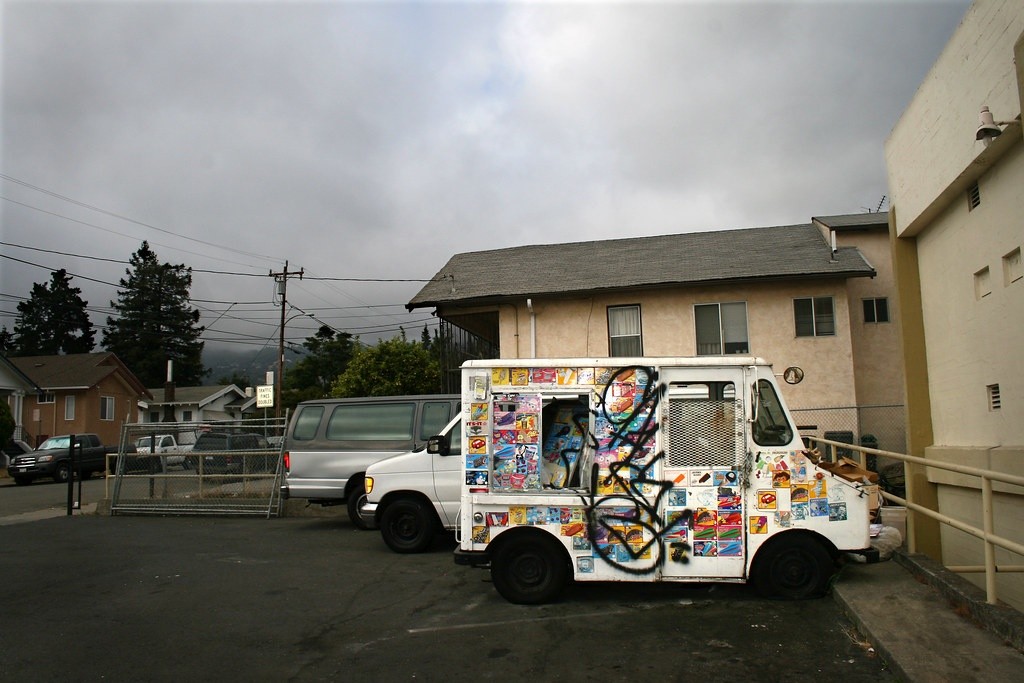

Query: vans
[451,355,871,606]
[359,410,464,555]
[281,393,462,530]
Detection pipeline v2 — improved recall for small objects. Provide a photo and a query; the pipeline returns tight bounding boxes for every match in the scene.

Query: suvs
[190,431,279,483]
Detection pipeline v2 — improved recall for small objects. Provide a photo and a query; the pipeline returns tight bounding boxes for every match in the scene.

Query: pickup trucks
[133,435,196,472]
[8,433,137,486]
